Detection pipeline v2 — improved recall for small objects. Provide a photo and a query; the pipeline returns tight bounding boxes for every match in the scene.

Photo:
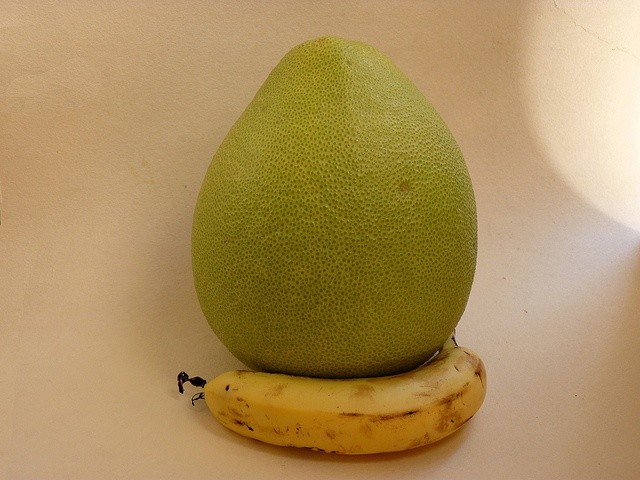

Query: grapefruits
[191,38,477,376]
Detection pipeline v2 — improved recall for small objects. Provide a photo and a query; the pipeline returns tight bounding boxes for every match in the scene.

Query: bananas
[204,327,485,456]
